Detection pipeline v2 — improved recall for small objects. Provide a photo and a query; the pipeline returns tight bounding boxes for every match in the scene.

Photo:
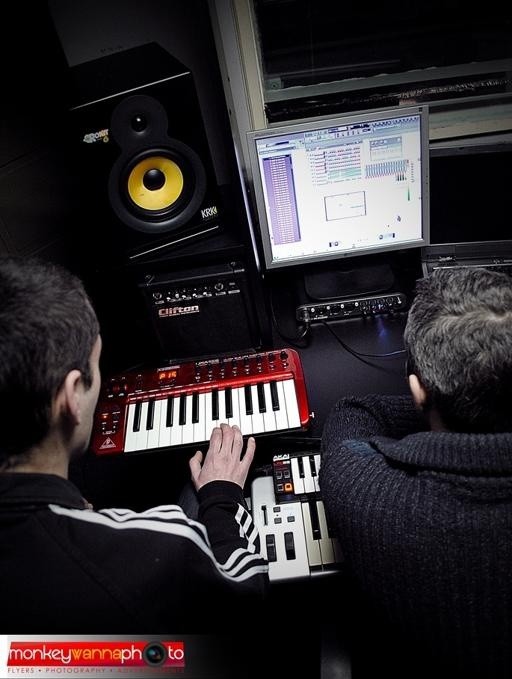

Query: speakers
[57,38,225,259]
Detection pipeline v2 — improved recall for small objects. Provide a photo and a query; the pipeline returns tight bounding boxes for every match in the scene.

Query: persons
[0,250,279,639]
[322,264,511,678]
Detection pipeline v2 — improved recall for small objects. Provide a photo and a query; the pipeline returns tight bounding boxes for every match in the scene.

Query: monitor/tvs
[245,102,431,300]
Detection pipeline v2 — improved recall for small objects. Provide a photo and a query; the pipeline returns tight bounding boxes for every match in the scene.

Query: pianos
[252,453,336,583]
[90,348,309,455]
